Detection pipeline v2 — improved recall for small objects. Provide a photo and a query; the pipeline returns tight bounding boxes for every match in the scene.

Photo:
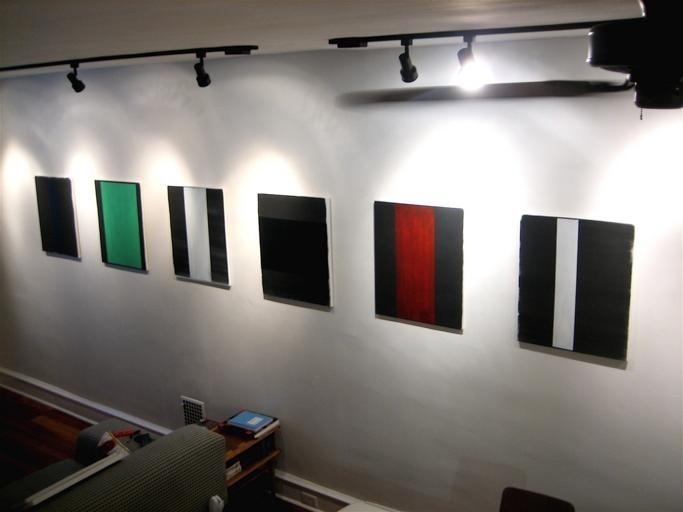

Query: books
[225,460,242,481]
[227,410,273,432]
[218,409,280,439]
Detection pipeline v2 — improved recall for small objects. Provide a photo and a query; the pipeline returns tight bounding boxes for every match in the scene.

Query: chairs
[0,413,228,512]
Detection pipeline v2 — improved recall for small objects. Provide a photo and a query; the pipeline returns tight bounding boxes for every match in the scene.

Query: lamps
[66,51,220,96]
[396,39,420,85]
